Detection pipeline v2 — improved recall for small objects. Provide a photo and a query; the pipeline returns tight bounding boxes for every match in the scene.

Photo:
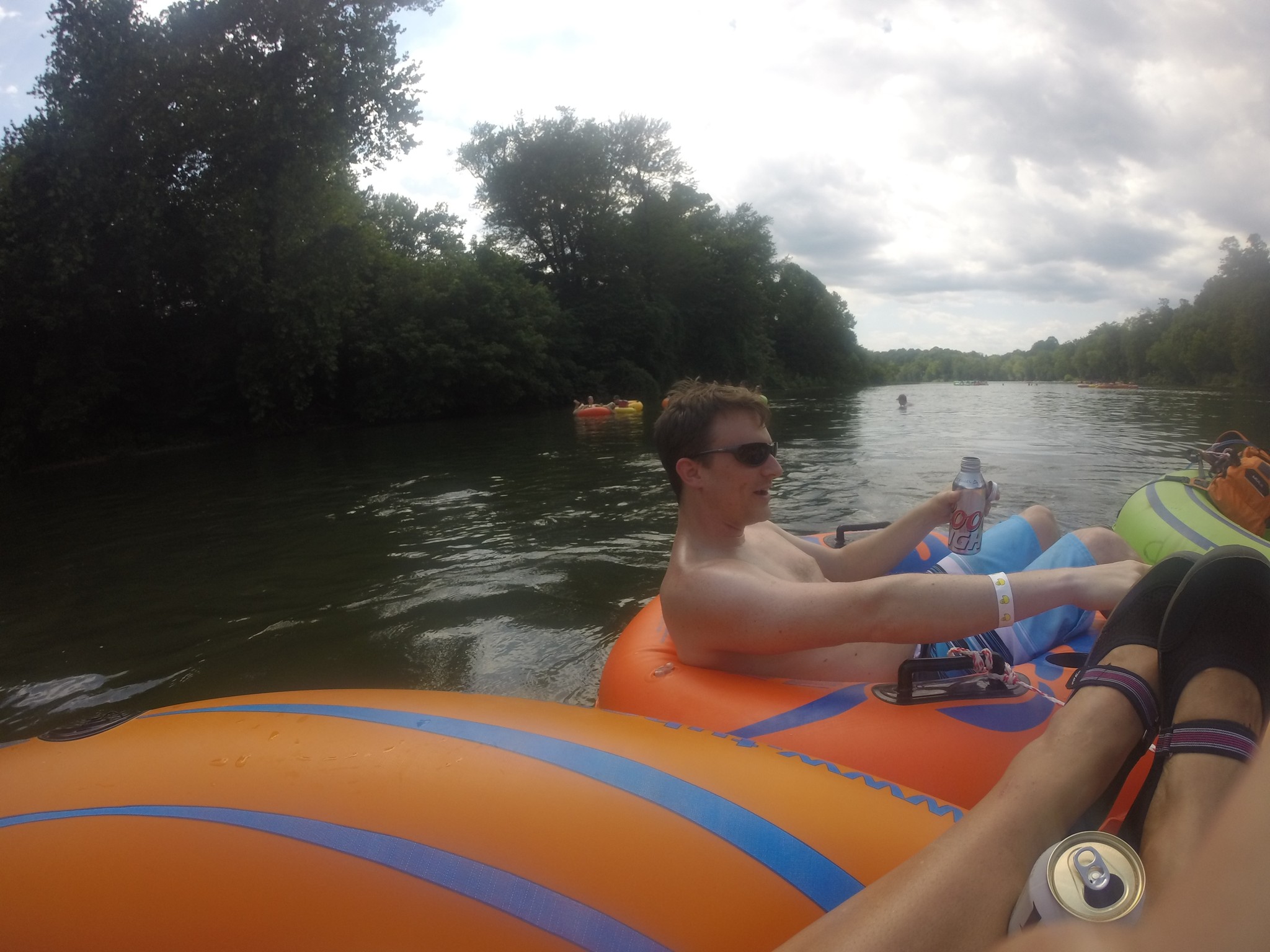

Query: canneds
[1008,831,1145,937]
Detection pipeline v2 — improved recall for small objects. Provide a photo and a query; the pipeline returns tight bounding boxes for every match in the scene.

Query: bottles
[947,456,987,555]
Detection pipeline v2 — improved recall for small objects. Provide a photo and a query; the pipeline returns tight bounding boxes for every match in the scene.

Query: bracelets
[988,571,1017,629]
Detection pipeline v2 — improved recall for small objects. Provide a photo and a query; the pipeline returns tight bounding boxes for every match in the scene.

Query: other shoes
[1062,544,1203,837]
[1116,545,1270,857]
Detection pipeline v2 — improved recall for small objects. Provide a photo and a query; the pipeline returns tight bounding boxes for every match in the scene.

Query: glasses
[687,442,778,466]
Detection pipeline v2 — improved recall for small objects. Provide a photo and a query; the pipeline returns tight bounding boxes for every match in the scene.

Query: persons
[773,544,1270,952]
[572,396,629,409]
[656,373,1150,684]
